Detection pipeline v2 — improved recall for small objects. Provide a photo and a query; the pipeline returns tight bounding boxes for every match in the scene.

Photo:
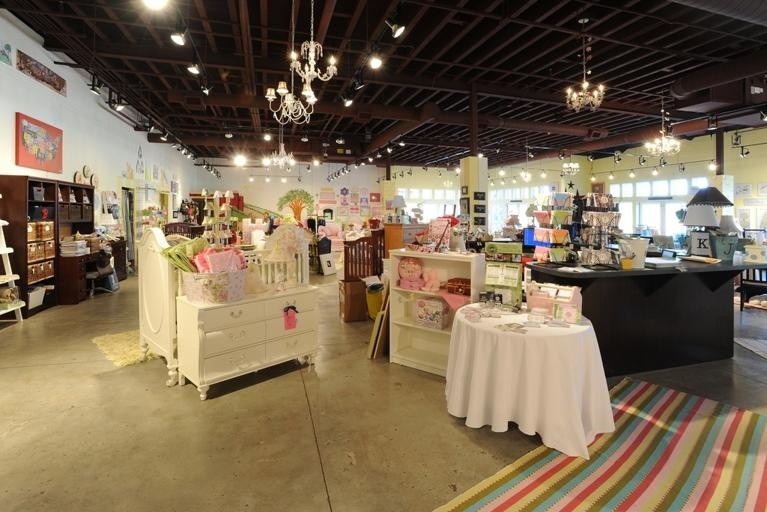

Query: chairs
[85,243,115,298]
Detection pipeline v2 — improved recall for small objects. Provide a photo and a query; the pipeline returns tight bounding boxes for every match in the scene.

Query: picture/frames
[462,186,468,194]
[757,183,767,195]
[756,207,767,230]
[474,205,486,213]
[474,217,485,225]
[1,39,14,68]
[15,112,65,175]
[734,183,752,196]
[459,198,470,214]
[735,208,752,239]
[474,192,485,200]
[15,48,68,98]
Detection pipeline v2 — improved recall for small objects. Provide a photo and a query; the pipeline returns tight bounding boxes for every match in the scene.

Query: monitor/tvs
[661,249,677,257]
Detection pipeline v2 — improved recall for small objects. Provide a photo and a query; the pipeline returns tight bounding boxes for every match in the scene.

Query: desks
[741,262,767,312]
[55,240,129,305]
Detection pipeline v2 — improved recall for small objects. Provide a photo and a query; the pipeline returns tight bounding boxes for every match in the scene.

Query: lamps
[683,204,720,256]
[561,154,580,176]
[644,68,680,158]
[391,195,406,223]
[709,215,744,261]
[506,214,521,240]
[564,17,607,113]
[270,119,297,170]
[686,187,733,234]
[265,0,317,127]
[288,0,339,85]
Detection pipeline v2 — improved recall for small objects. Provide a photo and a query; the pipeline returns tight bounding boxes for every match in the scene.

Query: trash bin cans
[365,283,384,321]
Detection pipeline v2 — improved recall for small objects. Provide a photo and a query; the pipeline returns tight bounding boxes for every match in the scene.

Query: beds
[133,222,311,387]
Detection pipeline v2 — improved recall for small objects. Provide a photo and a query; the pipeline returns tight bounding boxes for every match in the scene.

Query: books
[644,256,683,268]
[679,253,722,265]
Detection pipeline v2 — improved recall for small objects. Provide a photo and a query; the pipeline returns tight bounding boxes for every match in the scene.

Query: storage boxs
[181,268,247,305]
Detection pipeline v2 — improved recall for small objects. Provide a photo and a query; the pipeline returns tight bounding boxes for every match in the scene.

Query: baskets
[449,278,471,296]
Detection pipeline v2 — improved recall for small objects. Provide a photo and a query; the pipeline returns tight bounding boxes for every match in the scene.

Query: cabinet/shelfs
[445,301,616,460]
[388,249,485,376]
[530,191,623,264]
[0,174,95,320]
[175,285,321,401]
[384,225,429,258]
[525,262,747,377]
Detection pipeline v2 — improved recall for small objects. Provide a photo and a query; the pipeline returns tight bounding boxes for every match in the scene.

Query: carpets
[431,375,767,511]
[91,329,160,370]
